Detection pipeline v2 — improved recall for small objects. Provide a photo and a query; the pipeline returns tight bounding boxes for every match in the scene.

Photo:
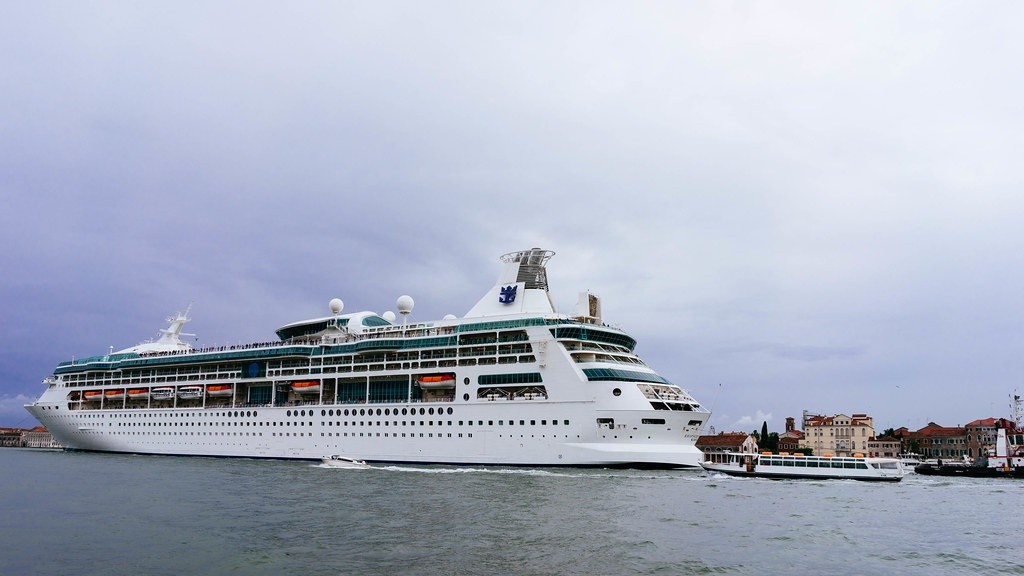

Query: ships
[21,247,713,469]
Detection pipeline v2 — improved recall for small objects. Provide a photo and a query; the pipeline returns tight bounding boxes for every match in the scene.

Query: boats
[418,375,456,390]
[176,386,205,400]
[701,451,904,482]
[151,388,175,401]
[128,389,149,399]
[289,381,320,394]
[105,390,124,398]
[84,392,101,402]
[206,386,234,397]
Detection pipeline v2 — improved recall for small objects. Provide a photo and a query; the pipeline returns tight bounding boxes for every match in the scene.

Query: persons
[937,457,942,468]
[139,337,317,357]
